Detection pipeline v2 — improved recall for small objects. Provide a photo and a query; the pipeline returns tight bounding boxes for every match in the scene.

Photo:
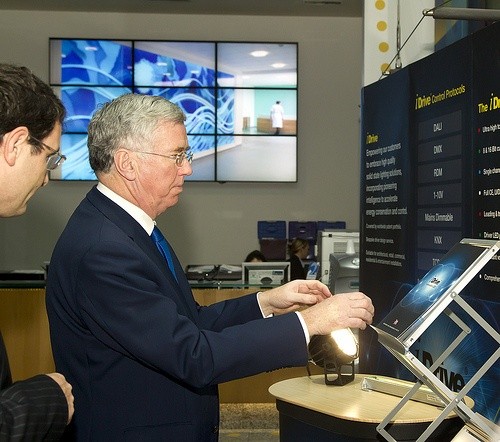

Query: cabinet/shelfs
[0,271,324,404]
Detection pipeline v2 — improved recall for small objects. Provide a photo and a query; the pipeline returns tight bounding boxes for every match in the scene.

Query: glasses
[27,133,67,170]
[121,146,194,167]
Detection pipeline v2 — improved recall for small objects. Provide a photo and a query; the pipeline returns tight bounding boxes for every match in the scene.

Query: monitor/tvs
[317,229,360,295]
[241,262,291,289]
[49,38,298,183]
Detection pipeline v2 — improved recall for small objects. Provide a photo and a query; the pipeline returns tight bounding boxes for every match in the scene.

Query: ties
[150,225,177,282]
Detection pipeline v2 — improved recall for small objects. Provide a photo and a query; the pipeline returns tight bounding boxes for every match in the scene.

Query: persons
[0,62,75,442]
[270,101,284,135]
[244,249,269,262]
[45,93,375,442]
[284,238,310,281]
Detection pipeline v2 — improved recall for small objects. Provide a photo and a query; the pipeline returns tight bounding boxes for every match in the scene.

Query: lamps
[305,325,358,385]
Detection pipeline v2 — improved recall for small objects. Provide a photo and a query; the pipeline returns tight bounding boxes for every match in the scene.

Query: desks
[269,374,476,442]
